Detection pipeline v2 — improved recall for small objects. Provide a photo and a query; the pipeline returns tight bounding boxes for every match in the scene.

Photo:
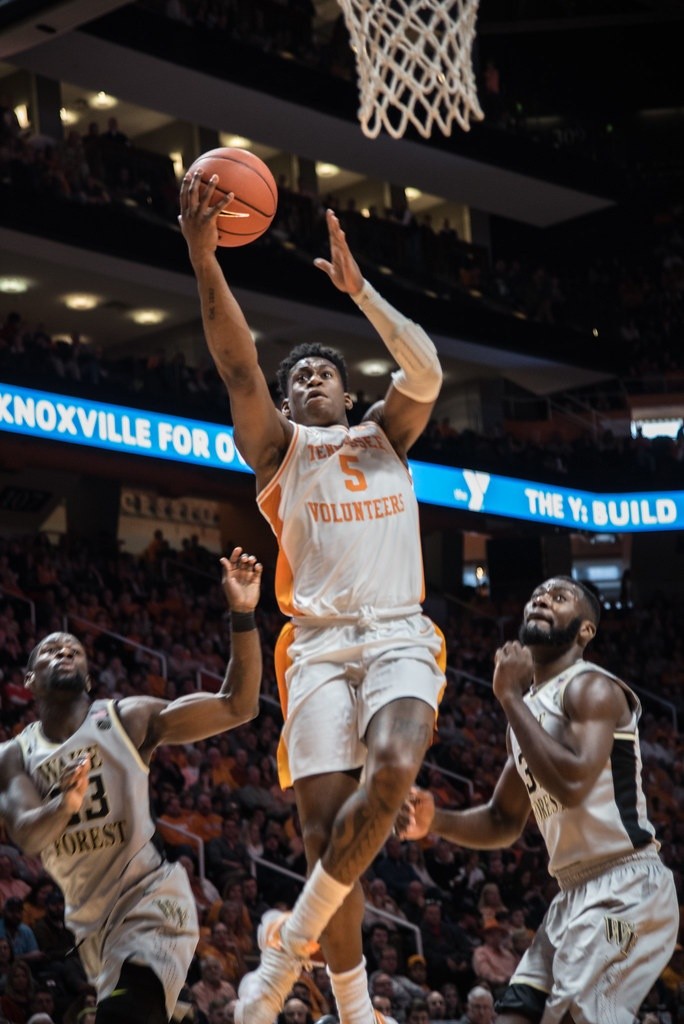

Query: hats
[407,956,427,968]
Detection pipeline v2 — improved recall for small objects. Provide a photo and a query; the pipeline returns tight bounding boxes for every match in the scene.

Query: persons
[177,169,446,1024]
[0,0,684,1024]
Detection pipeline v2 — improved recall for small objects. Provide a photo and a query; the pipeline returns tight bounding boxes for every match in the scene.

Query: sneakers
[233,908,320,1024]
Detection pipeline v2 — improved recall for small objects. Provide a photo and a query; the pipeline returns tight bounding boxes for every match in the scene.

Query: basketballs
[180,146,280,248]
